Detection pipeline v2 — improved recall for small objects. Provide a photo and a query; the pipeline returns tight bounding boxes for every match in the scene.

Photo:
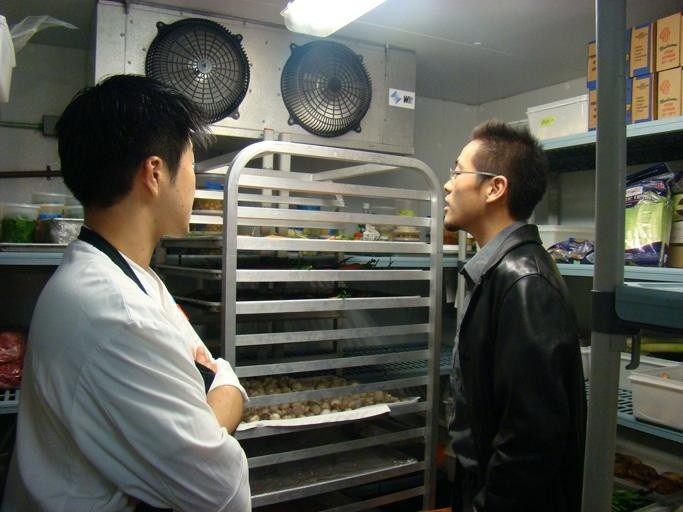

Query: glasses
[449,168,497,180]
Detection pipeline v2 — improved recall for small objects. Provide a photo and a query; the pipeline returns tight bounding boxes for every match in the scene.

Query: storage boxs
[575,315,683,432]
[527,12,681,139]
[535,223,595,258]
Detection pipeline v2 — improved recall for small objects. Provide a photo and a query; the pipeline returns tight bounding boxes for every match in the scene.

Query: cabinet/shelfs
[0,128,459,511]
[540,113,683,511]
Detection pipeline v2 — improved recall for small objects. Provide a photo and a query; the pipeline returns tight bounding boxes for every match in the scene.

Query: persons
[1,74,250,512]
[443,121,587,512]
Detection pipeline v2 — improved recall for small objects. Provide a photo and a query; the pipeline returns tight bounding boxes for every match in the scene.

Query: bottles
[358,203,370,233]
